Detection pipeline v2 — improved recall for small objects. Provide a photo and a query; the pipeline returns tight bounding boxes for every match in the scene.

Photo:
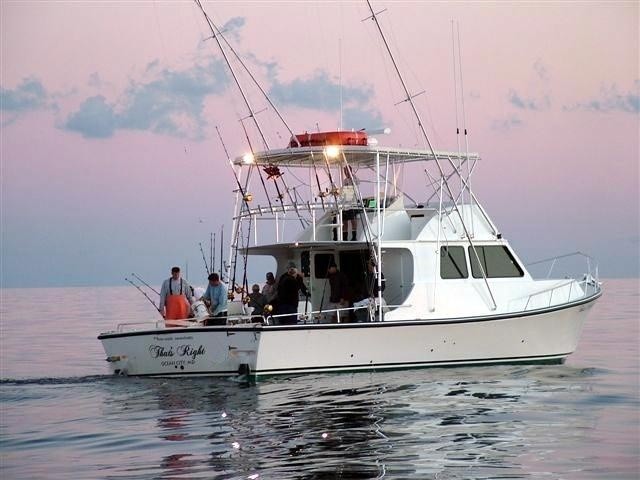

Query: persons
[199,274,227,326]
[160,268,194,328]
[326,263,346,323]
[358,260,385,323]
[330,166,359,240]
[245,261,311,325]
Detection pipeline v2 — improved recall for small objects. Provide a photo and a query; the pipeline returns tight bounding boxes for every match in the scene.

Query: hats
[252,285,259,289]
[266,272,273,279]
[287,262,295,268]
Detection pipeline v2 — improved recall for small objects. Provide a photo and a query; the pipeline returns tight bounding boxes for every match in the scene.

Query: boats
[99,3,602,388]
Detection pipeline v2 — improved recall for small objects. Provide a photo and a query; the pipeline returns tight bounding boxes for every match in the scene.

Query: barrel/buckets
[227,298,245,323]
[191,300,210,323]
